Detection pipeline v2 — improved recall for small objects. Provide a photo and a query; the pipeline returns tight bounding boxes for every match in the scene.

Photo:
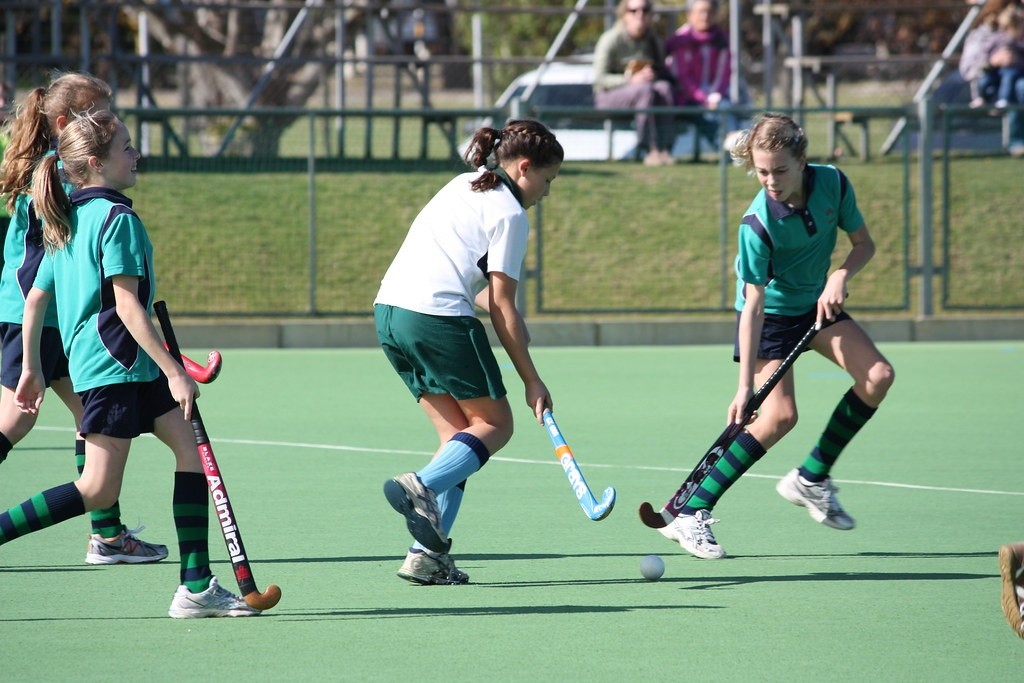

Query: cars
[455,54,754,163]
[896,65,1023,150]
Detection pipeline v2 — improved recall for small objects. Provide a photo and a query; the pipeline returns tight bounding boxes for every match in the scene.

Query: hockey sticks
[151,299,282,610]
[637,287,850,529]
[162,341,223,385]
[542,400,617,521]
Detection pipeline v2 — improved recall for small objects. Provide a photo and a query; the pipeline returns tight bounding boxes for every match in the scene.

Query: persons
[657,115,895,561]
[0,75,168,565]
[373,120,564,585]
[0,112,263,619]
[592,0,753,165]
[959,0,1024,156]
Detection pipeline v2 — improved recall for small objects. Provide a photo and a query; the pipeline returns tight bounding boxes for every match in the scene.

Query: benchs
[830,106,1018,164]
[559,114,752,163]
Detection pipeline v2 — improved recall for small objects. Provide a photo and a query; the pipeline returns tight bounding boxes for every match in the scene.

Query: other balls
[639,556,665,579]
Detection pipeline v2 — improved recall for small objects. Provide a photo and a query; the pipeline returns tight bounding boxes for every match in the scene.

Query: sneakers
[169,575,262,619]
[383,472,453,554]
[396,547,470,586]
[775,468,854,530]
[85,525,168,564]
[657,508,726,559]
